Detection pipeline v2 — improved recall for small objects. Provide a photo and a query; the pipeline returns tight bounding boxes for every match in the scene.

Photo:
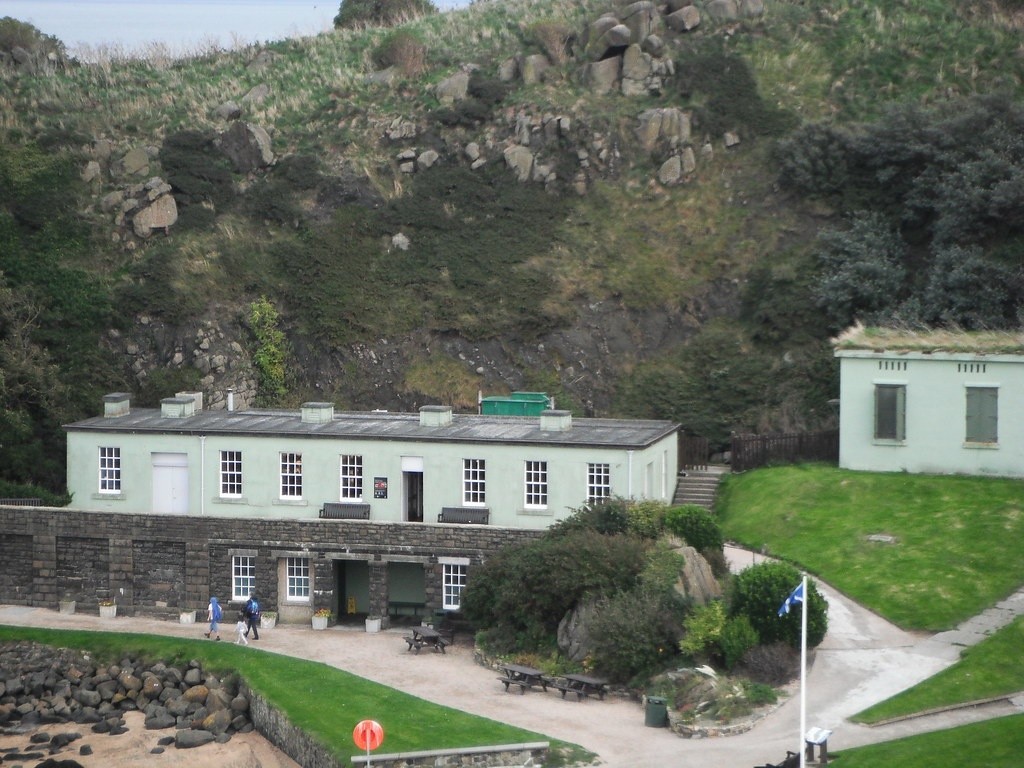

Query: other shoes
[243,641,248,646]
[234,641,239,644]
[252,638,259,640]
[204,633,210,638]
[216,636,220,641]
[243,633,247,637]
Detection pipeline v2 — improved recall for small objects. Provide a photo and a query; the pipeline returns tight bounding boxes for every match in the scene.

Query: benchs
[496,676,531,696]
[0,498,44,507]
[402,637,421,655]
[553,684,587,702]
[319,502,371,520]
[437,507,490,525]
[434,637,450,654]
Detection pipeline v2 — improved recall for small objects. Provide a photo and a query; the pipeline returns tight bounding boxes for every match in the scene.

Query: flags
[778,582,803,617]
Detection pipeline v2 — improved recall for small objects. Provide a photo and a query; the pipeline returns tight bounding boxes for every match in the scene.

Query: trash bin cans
[644,695,667,728]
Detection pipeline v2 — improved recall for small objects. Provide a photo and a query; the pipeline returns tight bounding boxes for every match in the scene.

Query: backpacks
[250,599,258,614]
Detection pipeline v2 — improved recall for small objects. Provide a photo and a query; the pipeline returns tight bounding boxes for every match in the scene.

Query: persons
[232,615,248,646]
[243,592,260,640]
[204,596,222,641]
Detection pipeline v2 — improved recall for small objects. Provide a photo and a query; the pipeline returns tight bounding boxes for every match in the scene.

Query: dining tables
[559,674,609,702]
[500,664,546,696]
[408,625,442,656]
